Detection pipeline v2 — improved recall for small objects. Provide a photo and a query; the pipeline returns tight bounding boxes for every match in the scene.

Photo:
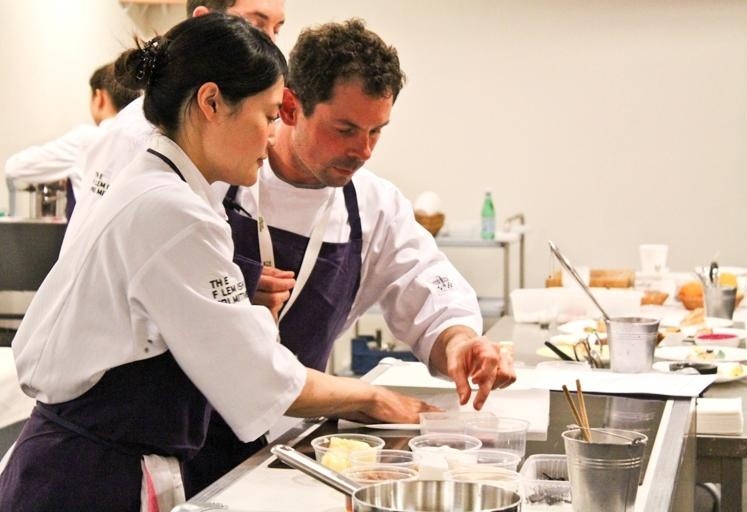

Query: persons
[183,19,517,505]
[1,64,140,220]
[56,1,288,260]
[0,12,445,511]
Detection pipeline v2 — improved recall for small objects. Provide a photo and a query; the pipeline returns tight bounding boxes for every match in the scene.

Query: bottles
[481,191,496,240]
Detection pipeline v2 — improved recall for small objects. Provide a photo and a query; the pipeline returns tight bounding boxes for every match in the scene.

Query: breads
[545,268,636,288]
[641,289,669,305]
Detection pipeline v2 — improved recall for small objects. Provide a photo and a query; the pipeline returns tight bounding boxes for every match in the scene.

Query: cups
[639,244,668,279]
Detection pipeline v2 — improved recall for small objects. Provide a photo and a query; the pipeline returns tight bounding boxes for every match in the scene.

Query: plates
[536,299,747,385]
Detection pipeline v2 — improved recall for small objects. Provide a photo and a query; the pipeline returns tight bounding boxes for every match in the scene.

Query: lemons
[677,273,736,297]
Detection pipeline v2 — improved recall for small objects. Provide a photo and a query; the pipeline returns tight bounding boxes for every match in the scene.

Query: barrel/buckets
[640,244,669,268]
[603,317,660,372]
[561,422,650,512]
[703,285,737,323]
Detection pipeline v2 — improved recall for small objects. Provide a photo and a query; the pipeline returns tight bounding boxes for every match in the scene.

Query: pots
[270,443,520,512]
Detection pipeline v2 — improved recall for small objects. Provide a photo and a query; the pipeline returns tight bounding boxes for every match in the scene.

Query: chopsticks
[560,379,592,444]
